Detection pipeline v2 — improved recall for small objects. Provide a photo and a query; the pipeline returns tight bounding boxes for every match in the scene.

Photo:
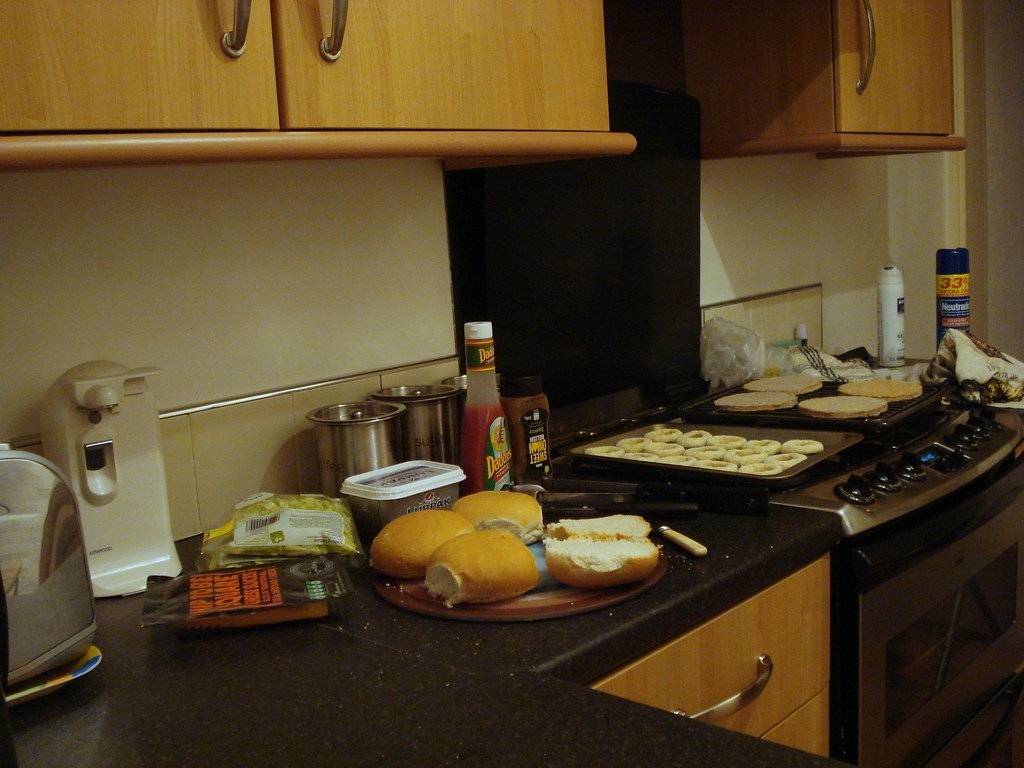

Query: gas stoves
[548,405,1024,543]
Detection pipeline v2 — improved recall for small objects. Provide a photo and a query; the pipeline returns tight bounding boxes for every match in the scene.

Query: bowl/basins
[339,458,468,543]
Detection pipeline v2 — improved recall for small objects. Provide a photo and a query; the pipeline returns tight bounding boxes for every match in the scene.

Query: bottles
[498,366,553,479]
[878,265,905,367]
[459,320,512,499]
[795,324,807,347]
[936,247,971,351]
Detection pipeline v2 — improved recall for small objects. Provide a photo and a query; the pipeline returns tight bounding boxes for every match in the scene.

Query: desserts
[585,428,824,477]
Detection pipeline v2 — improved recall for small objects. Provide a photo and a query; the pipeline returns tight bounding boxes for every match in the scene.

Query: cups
[305,404,405,498]
[367,385,460,465]
[440,374,500,413]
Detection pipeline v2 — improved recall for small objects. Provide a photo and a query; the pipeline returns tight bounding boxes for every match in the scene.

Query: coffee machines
[40,359,182,599]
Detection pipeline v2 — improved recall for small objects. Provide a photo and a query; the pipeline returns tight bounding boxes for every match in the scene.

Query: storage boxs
[337,458,467,541]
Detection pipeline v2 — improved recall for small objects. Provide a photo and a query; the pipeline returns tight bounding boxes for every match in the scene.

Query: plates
[4,644,102,707]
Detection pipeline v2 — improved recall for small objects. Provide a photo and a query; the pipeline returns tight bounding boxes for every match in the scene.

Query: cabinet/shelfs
[587,553,830,757]
[695,0,966,160]
[0,0,638,170]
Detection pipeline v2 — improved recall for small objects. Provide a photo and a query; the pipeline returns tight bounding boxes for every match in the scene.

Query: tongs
[500,470,770,517]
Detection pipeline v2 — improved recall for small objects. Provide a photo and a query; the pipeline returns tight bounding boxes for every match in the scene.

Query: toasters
[0,440,98,687]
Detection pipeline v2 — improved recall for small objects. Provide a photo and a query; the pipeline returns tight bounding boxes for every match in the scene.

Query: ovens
[831,460,1024,768]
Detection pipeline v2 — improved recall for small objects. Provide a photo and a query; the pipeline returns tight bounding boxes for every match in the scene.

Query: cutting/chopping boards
[375,521,669,621]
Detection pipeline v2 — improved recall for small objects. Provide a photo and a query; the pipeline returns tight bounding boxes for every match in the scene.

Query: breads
[370,491,660,610]
